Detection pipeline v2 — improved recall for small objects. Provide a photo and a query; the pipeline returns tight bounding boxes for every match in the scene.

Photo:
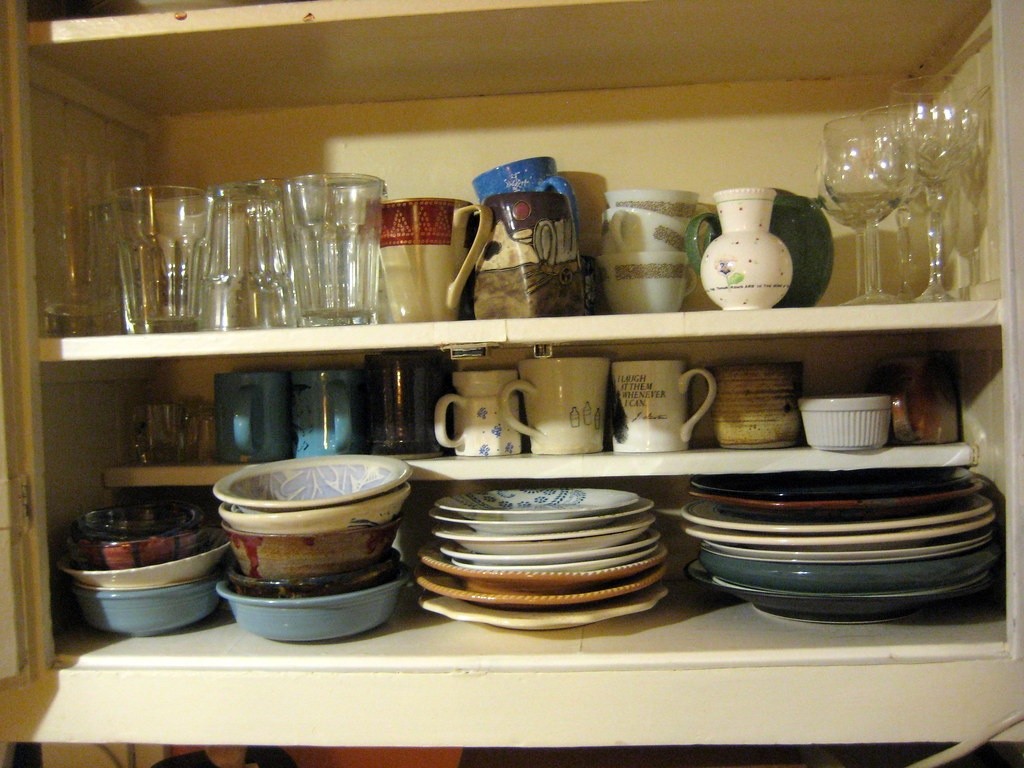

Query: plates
[417,474,1004,631]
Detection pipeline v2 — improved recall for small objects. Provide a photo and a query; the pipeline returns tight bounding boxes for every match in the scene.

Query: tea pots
[685,189,835,306]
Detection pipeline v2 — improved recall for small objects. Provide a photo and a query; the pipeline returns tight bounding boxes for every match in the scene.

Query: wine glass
[818,73,988,304]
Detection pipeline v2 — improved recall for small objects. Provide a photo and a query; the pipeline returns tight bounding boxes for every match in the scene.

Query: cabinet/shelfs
[1,1,1022,744]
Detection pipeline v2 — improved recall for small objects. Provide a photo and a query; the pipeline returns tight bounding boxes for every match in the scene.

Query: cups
[377,197,493,322]
[704,360,803,449]
[595,252,697,314]
[497,356,611,454]
[605,358,718,451]
[874,348,961,445]
[433,369,521,456]
[121,349,449,460]
[284,172,385,326]
[599,207,685,252]
[38,170,209,334]
[603,187,698,225]
[197,182,296,328]
[473,154,583,237]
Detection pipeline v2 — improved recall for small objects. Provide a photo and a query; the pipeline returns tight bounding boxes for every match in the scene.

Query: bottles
[472,189,586,317]
[700,188,793,309]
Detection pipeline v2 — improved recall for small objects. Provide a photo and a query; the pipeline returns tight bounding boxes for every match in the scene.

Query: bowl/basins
[50,453,413,642]
[799,394,891,451]
